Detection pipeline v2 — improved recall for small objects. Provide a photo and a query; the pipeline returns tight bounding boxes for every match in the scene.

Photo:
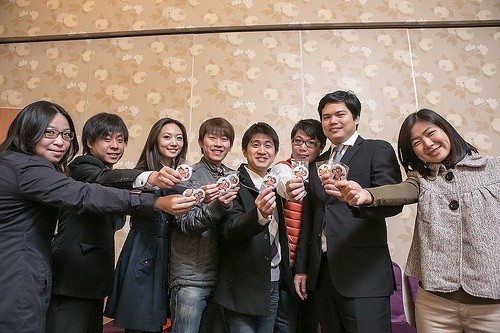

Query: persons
[293,90,405,333]
[177,164,347,203]
[271,119,328,333]
[46,113,181,333]
[170,118,239,333]
[103,118,220,333]
[0,100,196,333]
[334,108,500,333]
[213,122,293,333]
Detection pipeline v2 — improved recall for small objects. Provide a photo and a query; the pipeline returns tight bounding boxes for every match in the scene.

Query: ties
[333,145,344,163]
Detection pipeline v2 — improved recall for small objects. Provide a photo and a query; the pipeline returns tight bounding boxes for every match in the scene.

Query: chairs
[390,262,419,333]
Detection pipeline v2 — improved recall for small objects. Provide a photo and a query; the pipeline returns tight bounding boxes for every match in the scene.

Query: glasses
[291,138,321,148]
[43,129,74,141]
